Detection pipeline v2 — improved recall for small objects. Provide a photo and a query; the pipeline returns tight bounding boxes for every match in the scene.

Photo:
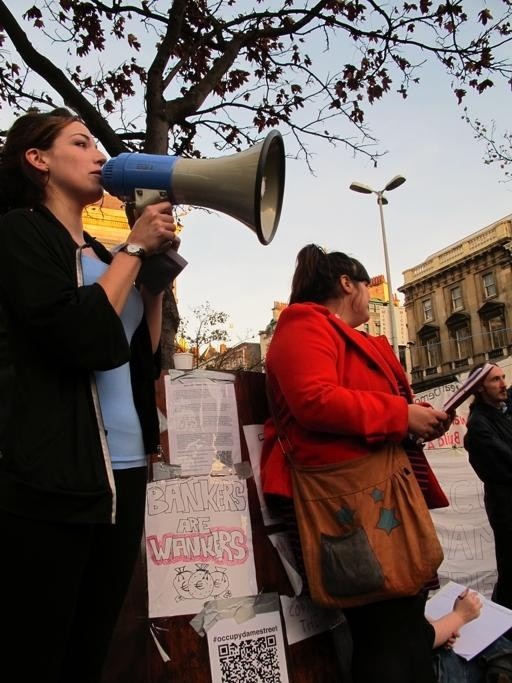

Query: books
[412,362,495,444]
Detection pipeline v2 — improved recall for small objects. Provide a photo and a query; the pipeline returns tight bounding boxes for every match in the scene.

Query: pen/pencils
[458,596,463,600]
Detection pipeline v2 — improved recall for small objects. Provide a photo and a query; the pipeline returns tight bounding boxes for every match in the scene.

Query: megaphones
[101,130,286,245]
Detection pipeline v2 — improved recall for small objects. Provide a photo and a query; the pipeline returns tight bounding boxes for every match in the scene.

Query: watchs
[123,241,147,257]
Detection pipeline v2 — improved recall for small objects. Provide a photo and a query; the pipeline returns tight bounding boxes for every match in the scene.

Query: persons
[259,245,447,681]
[352,584,482,682]
[464,363,511,610]
[0,110,180,680]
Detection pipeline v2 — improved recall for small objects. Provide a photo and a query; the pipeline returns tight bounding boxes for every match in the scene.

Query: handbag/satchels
[293,440,443,606]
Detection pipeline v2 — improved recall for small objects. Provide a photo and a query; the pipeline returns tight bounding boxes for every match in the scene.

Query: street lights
[346,176,406,382]
